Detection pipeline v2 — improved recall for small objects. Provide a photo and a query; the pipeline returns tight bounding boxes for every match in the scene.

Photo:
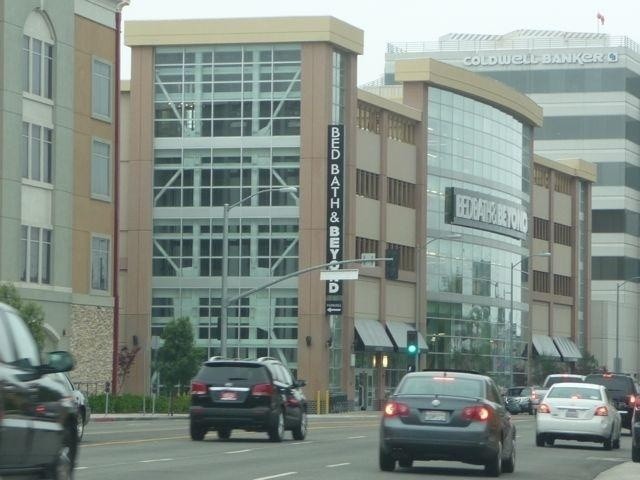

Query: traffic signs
[325,300,342,316]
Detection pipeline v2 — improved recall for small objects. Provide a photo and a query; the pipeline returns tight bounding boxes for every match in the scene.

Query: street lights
[220,186,298,354]
[415,234,463,369]
[510,253,552,386]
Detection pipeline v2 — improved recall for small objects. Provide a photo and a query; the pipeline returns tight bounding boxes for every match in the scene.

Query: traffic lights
[406,331,418,355]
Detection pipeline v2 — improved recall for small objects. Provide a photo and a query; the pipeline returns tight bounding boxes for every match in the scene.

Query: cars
[190,356,309,442]
[504,374,640,462]
[0,303,88,480]
[379,372,516,476]
